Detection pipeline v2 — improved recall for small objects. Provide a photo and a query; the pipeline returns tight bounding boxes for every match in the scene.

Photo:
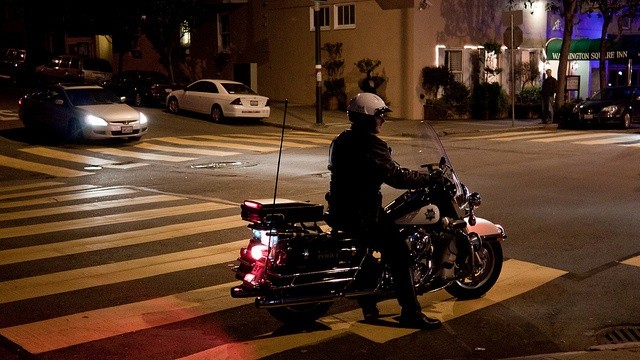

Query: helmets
[347,92,393,123]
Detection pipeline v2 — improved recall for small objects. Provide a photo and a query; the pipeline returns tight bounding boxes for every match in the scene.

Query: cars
[98,70,177,108]
[166,78,271,123]
[573,86,640,129]
[18,82,148,144]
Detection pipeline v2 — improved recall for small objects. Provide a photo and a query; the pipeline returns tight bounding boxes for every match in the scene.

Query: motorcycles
[228,121,507,323]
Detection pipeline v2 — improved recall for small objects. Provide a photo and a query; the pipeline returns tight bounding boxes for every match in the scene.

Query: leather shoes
[400,310,441,328]
[363,305,381,321]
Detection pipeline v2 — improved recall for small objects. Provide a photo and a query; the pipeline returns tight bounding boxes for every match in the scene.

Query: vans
[35,54,112,87]
[0,48,26,79]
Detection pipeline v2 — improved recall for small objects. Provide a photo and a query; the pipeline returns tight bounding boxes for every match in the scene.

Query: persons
[325,93,443,329]
[538,69,559,124]
